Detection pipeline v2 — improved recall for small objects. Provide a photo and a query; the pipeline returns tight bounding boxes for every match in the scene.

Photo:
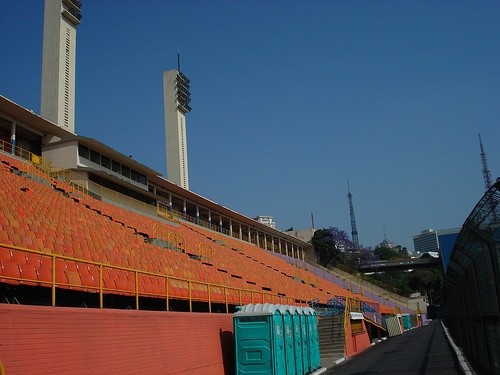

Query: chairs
[0,153,422,315]
